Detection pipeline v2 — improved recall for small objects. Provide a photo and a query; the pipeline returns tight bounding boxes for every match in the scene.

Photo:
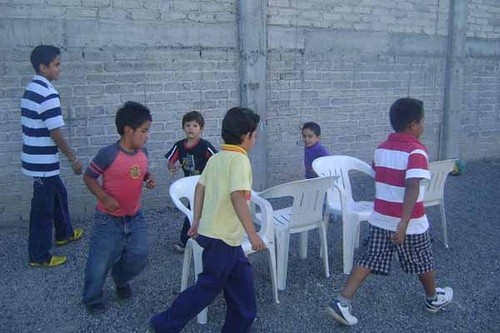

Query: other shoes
[28,255,67,267]
[89,303,105,316]
[118,284,132,299]
[56,227,84,245]
[174,240,184,252]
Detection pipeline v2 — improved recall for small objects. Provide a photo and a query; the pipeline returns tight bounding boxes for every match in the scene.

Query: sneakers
[327,298,358,326]
[424,286,454,313]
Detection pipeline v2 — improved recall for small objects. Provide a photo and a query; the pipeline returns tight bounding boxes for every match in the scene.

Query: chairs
[247,175,339,291]
[423,159,460,249]
[168,174,280,325]
[312,155,401,274]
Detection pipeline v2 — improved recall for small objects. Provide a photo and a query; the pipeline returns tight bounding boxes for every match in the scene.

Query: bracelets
[72,159,77,165]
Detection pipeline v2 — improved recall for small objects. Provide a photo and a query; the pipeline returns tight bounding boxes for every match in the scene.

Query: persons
[21,45,84,267]
[165,111,219,254]
[301,122,338,222]
[83,101,156,313]
[149,107,265,333]
[326,98,453,325]
